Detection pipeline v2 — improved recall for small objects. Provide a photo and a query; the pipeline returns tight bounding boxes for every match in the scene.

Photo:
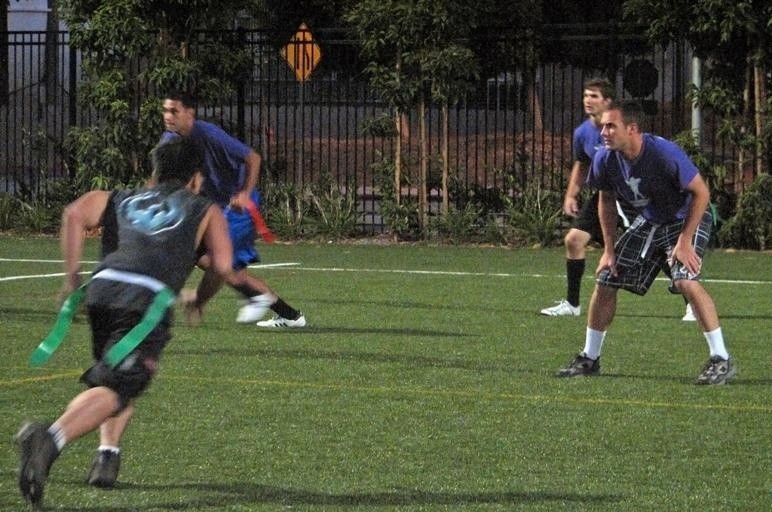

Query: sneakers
[693,354,738,386]
[682,303,699,321]
[540,298,582,317]
[554,352,602,379]
[14,423,62,507]
[235,291,274,324]
[257,310,307,329]
[84,448,122,489]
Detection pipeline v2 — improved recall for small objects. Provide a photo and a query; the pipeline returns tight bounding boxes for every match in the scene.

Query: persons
[151,91,306,327]
[14,139,244,512]
[556,97,738,388]
[540,74,697,323]
